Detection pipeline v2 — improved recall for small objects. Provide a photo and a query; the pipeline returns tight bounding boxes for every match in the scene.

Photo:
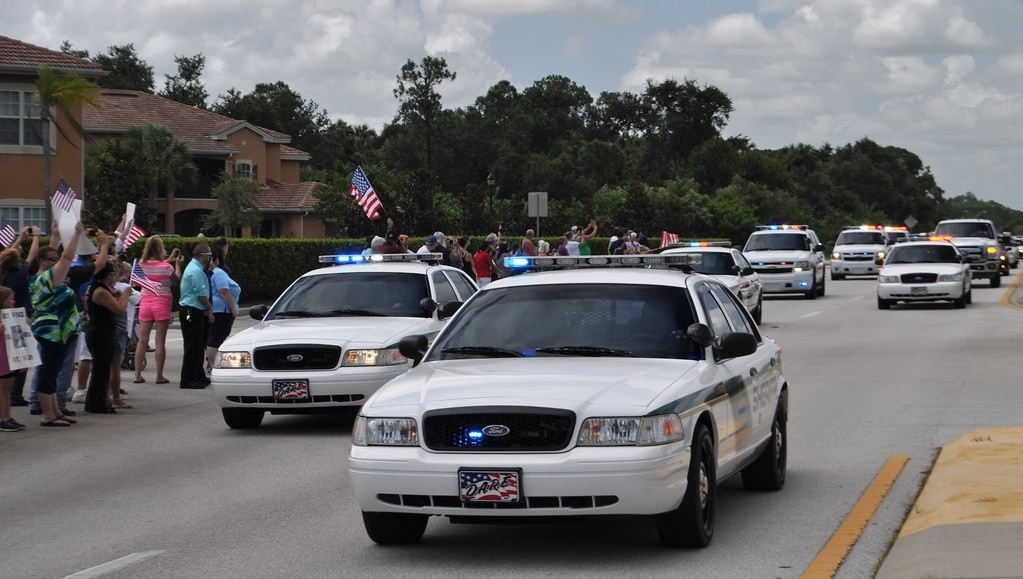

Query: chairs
[675,298,695,331]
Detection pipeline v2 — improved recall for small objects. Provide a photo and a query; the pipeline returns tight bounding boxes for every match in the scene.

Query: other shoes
[180,376,211,389]
[60,407,76,415]
[11,397,30,407]
[40,414,77,427]
[30,408,46,415]
[102,404,117,414]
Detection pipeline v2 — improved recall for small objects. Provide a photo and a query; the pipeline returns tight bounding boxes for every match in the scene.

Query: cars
[741,223,827,299]
[210,251,481,429]
[348,254,788,548]
[830,218,1023,287]
[876,237,973,309]
[648,239,763,326]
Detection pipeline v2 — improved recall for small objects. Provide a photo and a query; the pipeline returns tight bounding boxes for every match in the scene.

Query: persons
[0,214,241,431]
[363,217,650,289]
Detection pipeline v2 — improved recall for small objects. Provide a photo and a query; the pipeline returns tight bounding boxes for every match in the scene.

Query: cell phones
[93,228,98,236]
[27,228,32,233]
[175,249,180,256]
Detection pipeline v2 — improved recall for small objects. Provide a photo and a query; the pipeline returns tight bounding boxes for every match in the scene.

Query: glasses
[200,252,212,256]
[47,256,59,263]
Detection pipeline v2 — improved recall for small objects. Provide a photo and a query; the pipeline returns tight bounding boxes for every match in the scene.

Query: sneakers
[71,388,88,403]
[65,386,76,400]
[0,418,26,432]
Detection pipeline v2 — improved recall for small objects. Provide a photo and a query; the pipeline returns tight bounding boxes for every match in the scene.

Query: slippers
[133,377,145,383]
[155,378,170,383]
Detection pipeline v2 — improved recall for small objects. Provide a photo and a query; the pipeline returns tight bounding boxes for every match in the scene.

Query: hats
[426,235,437,243]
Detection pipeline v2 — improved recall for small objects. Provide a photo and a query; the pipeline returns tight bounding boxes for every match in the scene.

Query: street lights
[487,173,496,234]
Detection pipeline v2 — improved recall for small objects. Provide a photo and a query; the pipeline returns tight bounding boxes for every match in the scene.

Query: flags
[348,165,384,220]
[0,223,16,247]
[131,263,161,297]
[51,176,76,213]
[122,224,147,252]
